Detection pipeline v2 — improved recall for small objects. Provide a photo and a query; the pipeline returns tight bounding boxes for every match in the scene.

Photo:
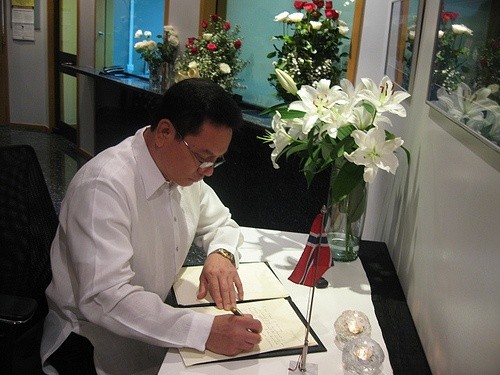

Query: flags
[288,213,334,288]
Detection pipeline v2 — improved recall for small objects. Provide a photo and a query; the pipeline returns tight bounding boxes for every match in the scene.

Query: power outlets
[127,64,134,72]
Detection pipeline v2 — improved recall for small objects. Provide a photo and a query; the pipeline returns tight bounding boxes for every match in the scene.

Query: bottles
[161,61,172,90]
[325,165,367,261]
[150,65,160,92]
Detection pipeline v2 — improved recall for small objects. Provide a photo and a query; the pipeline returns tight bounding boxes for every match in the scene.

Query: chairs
[0,145,60,375]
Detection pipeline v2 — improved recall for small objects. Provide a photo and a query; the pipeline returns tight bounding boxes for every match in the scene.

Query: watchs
[215,248,235,266]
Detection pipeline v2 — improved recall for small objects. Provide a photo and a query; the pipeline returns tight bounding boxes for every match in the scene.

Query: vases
[325,165,368,262]
[149,62,170,91]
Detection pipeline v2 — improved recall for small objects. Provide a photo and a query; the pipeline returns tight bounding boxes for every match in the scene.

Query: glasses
[178,134,226,169]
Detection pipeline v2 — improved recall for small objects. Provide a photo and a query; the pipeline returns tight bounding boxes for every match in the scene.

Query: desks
[155,227,433,375]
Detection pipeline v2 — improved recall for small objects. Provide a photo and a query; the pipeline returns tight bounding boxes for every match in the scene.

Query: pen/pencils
[231,306,243,318]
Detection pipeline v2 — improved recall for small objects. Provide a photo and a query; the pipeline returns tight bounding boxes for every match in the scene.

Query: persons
[38,78,263,375]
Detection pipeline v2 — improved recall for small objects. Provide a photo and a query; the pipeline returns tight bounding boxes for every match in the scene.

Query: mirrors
[426,0,500,154]
[384,0,424,98]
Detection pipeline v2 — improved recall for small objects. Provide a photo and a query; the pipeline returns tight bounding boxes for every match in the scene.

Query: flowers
[266,0,352,101]
[402,15,417,91]
[256,68,412,222]
[172,13,252,93]
[432,10,500,141]
[134,24,180,62]
